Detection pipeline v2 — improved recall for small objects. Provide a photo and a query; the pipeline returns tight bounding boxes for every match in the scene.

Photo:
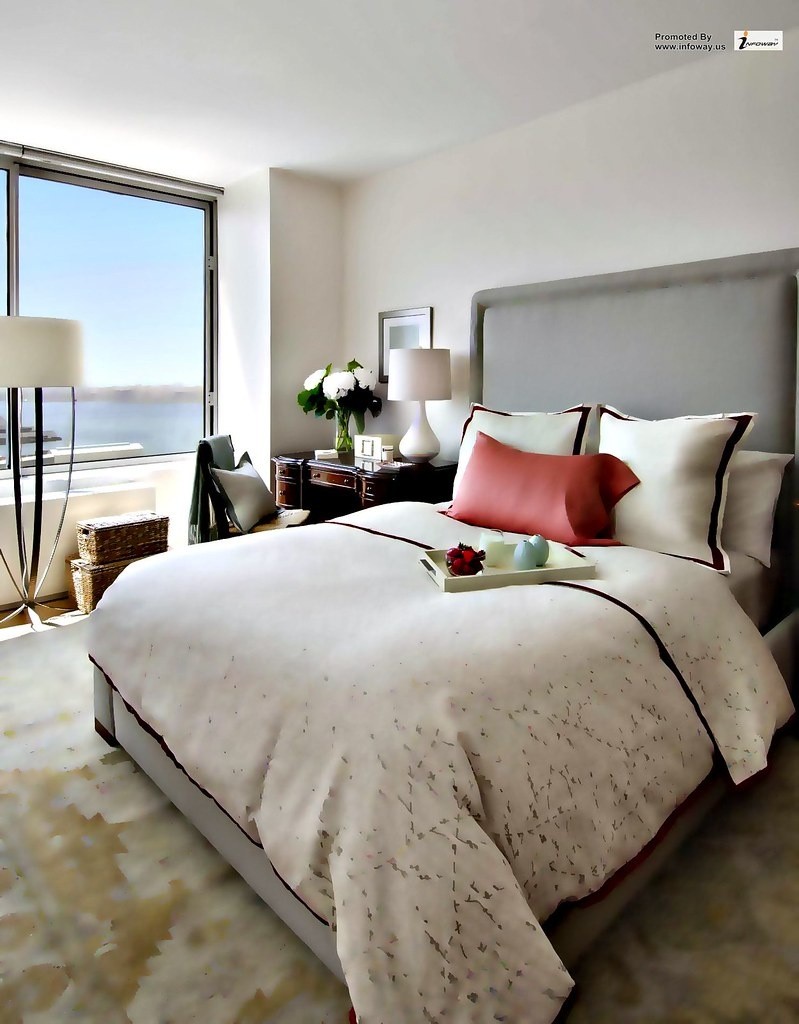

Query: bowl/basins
[444,553,485,577]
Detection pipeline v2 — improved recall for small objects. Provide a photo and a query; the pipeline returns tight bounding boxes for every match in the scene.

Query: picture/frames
[377,305,433,383]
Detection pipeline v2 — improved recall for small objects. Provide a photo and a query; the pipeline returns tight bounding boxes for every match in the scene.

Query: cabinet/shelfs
[271,447,459,527]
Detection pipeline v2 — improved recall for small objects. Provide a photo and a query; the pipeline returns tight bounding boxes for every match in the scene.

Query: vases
[334,409,351,452]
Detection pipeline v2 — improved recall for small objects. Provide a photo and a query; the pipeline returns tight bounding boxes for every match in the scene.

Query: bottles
[513,540,537,571]
[382,446,393,464]
[529,534,549,566]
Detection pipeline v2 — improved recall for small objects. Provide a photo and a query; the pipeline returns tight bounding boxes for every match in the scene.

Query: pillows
[206,451,286,535]
[719,450,794,569]
[451,401,594,507]
[596,402,756,577]
[436,429,641,548]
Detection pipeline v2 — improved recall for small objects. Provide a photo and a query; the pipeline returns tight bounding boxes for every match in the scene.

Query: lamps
[0,314,84,632]
[387,348,453,465]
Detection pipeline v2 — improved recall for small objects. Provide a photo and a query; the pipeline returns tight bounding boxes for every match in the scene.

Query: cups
[478,529,504,566]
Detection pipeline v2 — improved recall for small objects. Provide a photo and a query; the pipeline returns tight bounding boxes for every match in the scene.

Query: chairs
[196,433,310,540]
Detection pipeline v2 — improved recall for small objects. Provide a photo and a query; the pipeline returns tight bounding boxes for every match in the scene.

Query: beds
[82,496,799,1024]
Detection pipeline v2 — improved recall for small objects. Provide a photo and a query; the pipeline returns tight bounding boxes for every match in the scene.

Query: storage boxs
[75,510,169,567]
[353,432,403,461]
[64,553,159,616]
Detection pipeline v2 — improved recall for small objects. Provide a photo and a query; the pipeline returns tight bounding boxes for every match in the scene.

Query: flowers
[296,357,384,452]
